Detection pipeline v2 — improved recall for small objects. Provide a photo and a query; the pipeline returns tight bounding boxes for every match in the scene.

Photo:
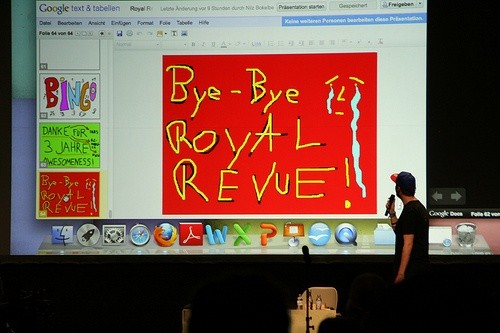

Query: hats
[390,171,416,190]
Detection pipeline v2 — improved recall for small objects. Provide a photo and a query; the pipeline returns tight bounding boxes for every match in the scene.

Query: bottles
[297,294,304,310]
[316,294,322,310]
[308,292,312,309]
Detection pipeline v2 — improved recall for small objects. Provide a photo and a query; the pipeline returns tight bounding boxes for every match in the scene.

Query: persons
[0,268,500,333]
[385,171,429,284]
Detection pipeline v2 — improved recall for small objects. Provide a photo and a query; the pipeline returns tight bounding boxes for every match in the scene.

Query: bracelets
[390,212,395,217]
[392,222,397,225]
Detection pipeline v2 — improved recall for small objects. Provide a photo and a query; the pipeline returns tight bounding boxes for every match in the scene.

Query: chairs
[302,287,339,310]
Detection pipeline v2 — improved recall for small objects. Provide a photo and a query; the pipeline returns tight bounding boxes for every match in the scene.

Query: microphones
[302,246,311,273]
[385,194,395,216]
